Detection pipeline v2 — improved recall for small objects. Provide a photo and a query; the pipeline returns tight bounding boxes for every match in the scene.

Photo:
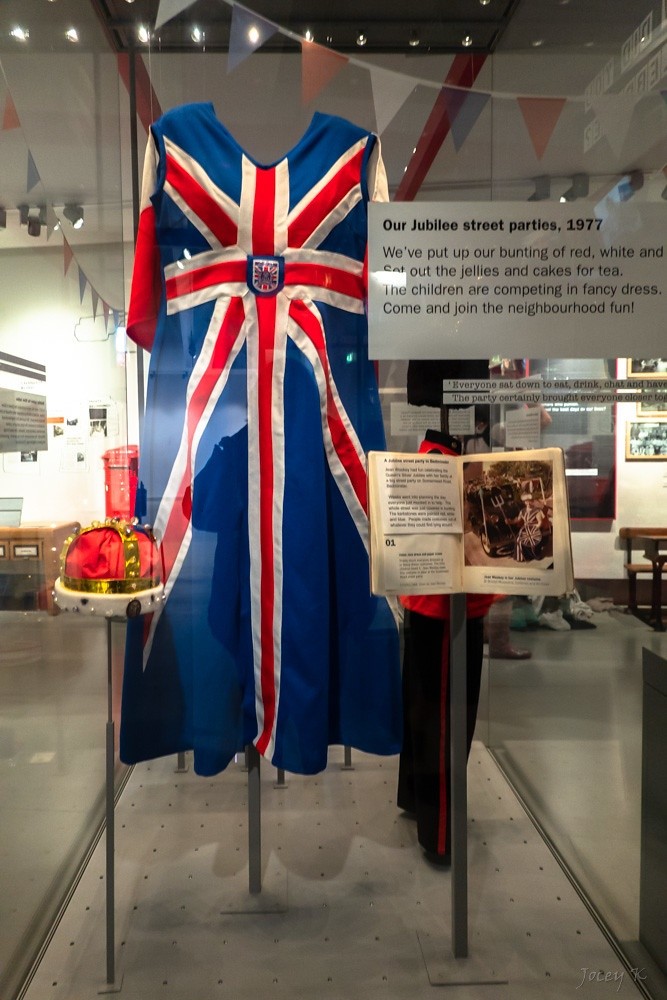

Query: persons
[505,494,543,561]
[398,429,496,869]
[490,388,554,449]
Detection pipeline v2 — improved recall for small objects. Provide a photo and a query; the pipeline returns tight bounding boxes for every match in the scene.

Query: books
[367,447,575,596]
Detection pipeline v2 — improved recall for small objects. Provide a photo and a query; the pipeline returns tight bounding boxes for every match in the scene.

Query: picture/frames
[625,418,667,460]
[626,358,667,380]
[634,384,667,418]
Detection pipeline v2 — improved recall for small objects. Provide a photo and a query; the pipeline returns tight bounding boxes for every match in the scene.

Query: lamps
[526,176,551,200]
[560,174,590,203]
[17,204,41,236]
[618,170,642,198]
[62,206,83,229]
[39,205,60,231]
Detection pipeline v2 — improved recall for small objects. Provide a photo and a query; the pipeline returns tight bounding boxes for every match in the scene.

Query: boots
[489,600,532,658]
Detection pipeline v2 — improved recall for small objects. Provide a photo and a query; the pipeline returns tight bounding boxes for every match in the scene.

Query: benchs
[619,525,667,622]
[0,520,82,616]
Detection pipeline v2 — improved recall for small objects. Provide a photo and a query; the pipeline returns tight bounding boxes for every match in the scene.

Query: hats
[52,517,165,616]
[521,491,532,501]
